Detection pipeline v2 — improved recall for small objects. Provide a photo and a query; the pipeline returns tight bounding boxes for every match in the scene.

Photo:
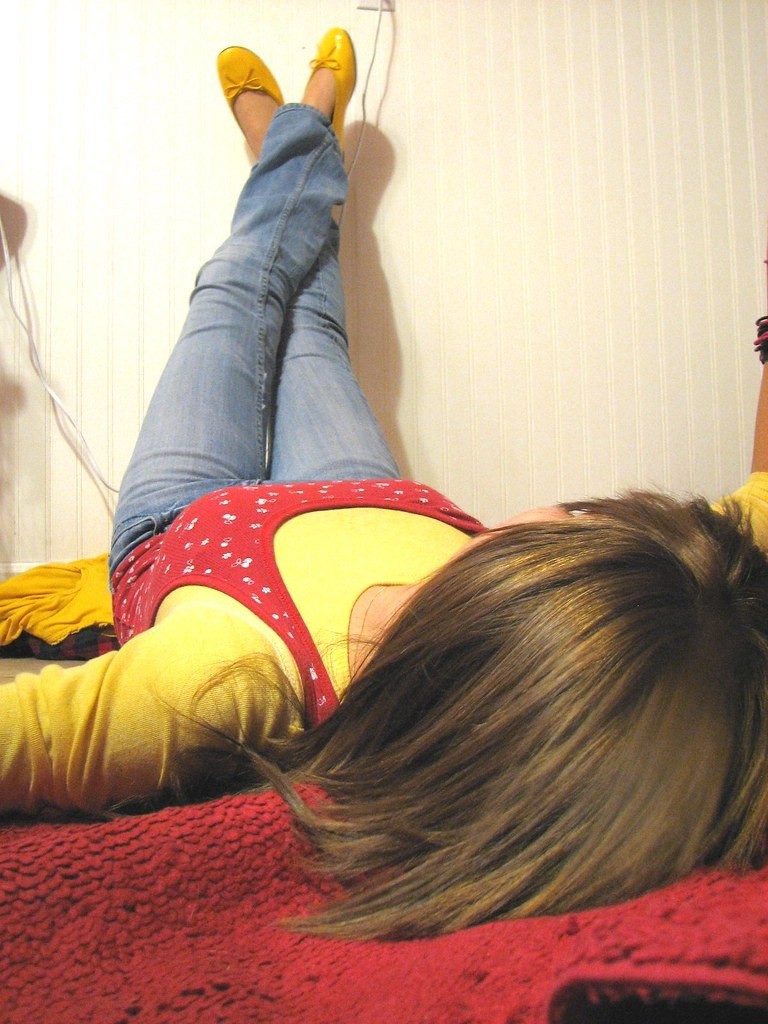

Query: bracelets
[751,314,767,365]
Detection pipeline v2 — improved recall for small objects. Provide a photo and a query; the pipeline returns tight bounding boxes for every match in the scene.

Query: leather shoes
[305,27,356,162]
[216,46,286,149]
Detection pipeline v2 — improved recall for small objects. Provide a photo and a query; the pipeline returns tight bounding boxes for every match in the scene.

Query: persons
[0,27,768,941]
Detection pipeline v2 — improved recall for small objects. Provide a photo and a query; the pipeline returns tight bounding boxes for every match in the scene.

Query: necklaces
[352,586,386,676]
